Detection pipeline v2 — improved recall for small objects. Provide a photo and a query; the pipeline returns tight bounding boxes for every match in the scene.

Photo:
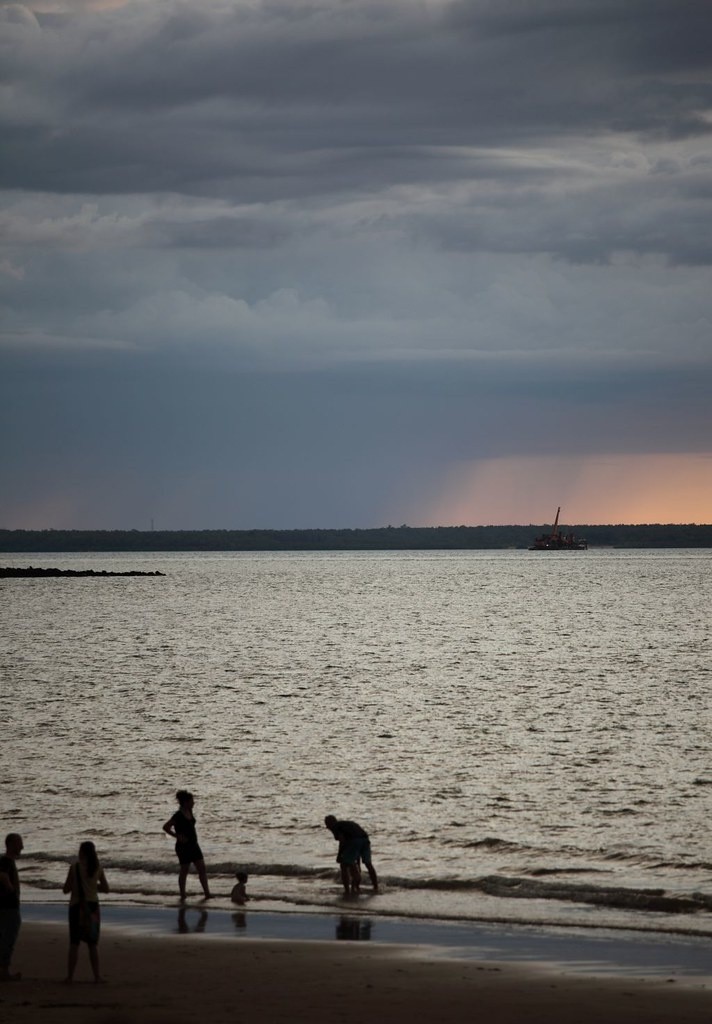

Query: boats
[527,507,588,550]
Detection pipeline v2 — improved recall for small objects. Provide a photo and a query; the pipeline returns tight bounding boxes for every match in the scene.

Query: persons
[163,791,214,898]
[63,842,110,982]
[0,832,23,981]
[232,872,249,904]
[324,815,378,898]
